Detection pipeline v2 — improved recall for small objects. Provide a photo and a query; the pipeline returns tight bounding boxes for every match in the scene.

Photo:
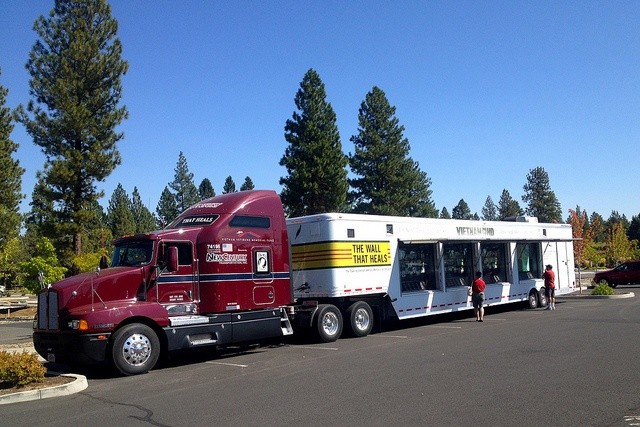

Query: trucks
[33,190,587,376]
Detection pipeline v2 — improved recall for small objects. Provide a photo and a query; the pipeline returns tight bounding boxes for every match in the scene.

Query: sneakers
[552,306,555,310]
[544,306,552,310]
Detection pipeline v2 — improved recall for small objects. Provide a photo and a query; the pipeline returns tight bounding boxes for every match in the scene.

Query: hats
[545,263,553,268]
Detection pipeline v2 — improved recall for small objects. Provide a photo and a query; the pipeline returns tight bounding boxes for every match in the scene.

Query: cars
[593,260,640,288]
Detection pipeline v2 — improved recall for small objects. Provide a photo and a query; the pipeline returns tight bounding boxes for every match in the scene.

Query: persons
[471,271,486,323]
[616,259,625,270]
[541,264,555,311]
